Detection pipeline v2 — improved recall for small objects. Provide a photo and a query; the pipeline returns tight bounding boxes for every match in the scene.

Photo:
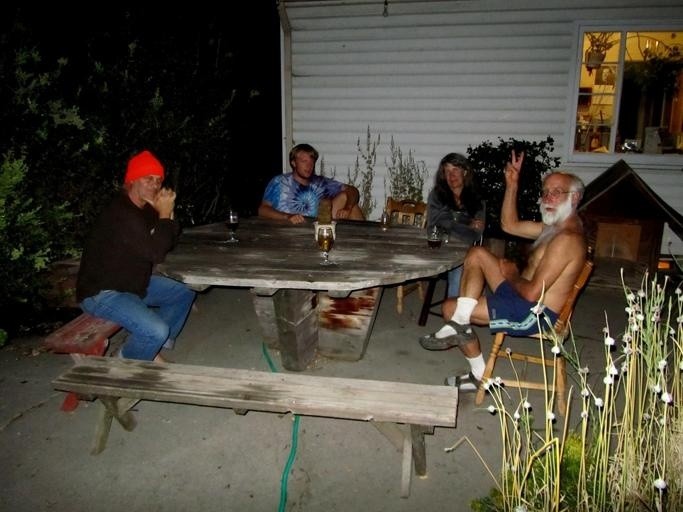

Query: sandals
[444,370,481,394]
[419,320,476,350]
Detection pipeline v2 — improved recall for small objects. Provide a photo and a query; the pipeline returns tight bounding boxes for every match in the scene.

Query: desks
[158,217,479,372]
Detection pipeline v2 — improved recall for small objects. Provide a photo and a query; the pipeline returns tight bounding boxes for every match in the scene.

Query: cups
[426,225,442,248]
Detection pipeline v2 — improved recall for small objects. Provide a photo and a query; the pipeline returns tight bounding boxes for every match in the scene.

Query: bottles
[381,209,389,232]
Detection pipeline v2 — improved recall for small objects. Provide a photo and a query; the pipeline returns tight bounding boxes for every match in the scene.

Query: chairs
[385,196,429,314]
[417,200,486,327]
[474,247,594,417]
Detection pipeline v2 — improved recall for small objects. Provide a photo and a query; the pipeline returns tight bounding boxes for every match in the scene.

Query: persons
[76,151,194,361]
[420,150,586,392]
[425,152,494,298]
[596,66,614,85]
[258,144,363,224]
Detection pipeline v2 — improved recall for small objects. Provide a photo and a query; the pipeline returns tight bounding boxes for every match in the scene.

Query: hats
[124,149,165,187]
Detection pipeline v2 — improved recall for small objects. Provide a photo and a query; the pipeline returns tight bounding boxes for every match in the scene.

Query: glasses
[538,189,568,201]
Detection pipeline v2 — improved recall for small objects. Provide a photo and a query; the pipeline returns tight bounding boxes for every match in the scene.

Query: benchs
[45,312,122,412]
[50,354,459,500]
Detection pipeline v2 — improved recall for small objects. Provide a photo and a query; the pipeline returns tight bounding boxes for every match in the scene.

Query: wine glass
[318,227,335,266]
[225,210,239,242]
